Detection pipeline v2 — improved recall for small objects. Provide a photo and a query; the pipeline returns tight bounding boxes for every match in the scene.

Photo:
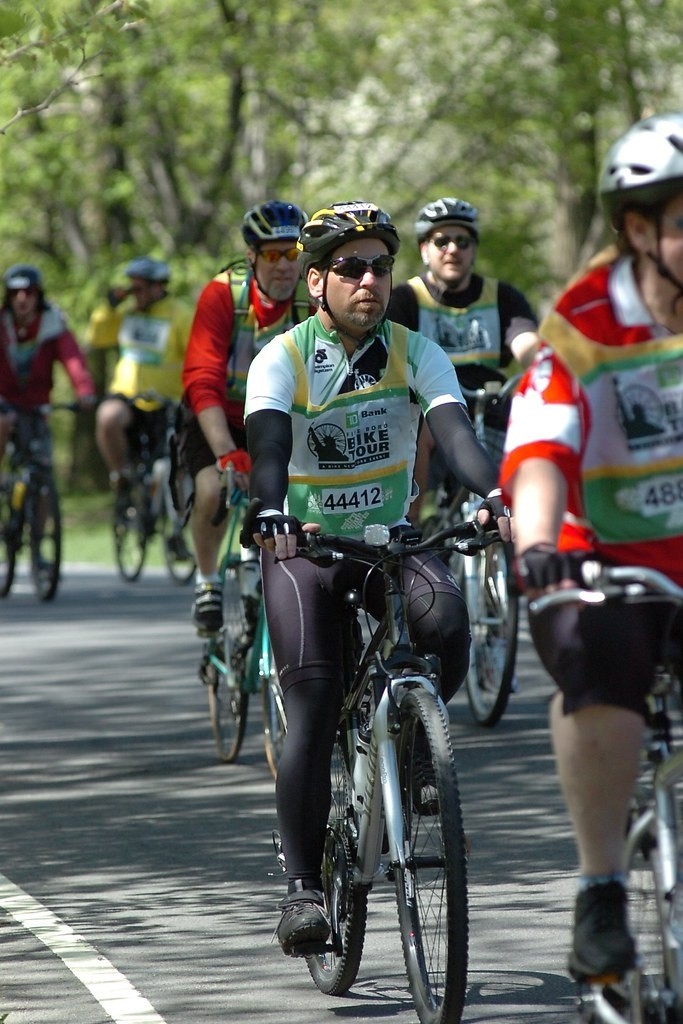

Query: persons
[387,199,537,531]
[494,114,683,984]
[86,258,193,556]
[241,202,518,955]
[172,201,317,695]
[0,265,97,577]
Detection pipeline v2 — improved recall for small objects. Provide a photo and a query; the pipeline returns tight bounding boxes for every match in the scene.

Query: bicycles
[112,395,199,585]
[261,511,504,1024]
[529,565,683,1024]
[0,401,83,604]
[200,483,279,778]
[424,369,521,724]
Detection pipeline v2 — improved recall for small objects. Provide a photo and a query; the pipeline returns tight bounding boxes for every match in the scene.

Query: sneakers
[400,757,440,817]
[565,870,637,982]
[192,581,224,628]
[271,890,332,957]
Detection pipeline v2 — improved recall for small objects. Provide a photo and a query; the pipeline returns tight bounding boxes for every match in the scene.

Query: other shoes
[167,535,192,562]
[115,484,133,517]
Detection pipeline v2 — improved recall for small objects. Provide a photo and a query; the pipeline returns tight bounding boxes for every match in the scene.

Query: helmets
[125,258,169,282]
[3,264,41,290]
[240,200,308,250]
[296,200,401,281]
[415,197,480,248]
[599,111,683,256]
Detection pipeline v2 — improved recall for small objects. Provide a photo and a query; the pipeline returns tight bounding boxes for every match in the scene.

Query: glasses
[125,284,144,295]
[258,248,298,263]
[661,214,683,232]
[327,255,395,279]
[10,289,33,295]
[429,234,473,249]
